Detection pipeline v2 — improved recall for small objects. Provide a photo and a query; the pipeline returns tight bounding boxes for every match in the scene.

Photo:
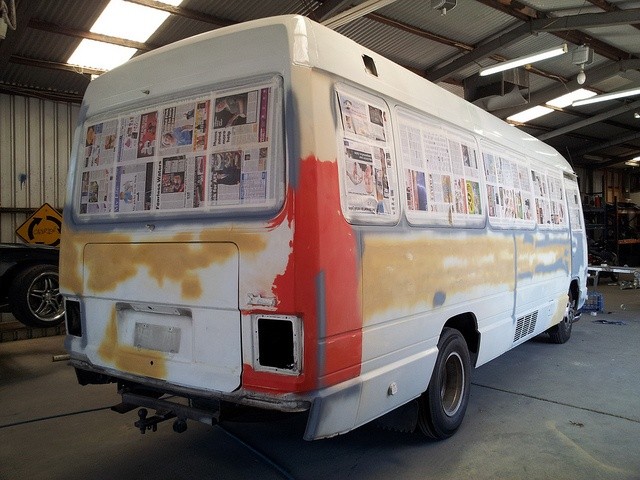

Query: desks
[588,265,640,288]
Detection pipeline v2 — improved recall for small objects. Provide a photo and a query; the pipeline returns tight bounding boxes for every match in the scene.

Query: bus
[53,15,588,441]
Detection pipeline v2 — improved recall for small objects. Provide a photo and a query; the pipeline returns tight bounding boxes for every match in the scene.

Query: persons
[354,163,375,195]
[215,96,246,118]
[162,124,194,145]
[85,126,94,145]
[170,174,183,192]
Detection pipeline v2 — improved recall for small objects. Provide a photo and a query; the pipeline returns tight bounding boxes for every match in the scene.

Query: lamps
[479,43,569,78]
[576,63,587,86]
[571,87,640,108]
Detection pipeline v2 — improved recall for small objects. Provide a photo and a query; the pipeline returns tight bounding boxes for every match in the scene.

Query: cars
[586,235,620,282]
[0,242,65,328]
